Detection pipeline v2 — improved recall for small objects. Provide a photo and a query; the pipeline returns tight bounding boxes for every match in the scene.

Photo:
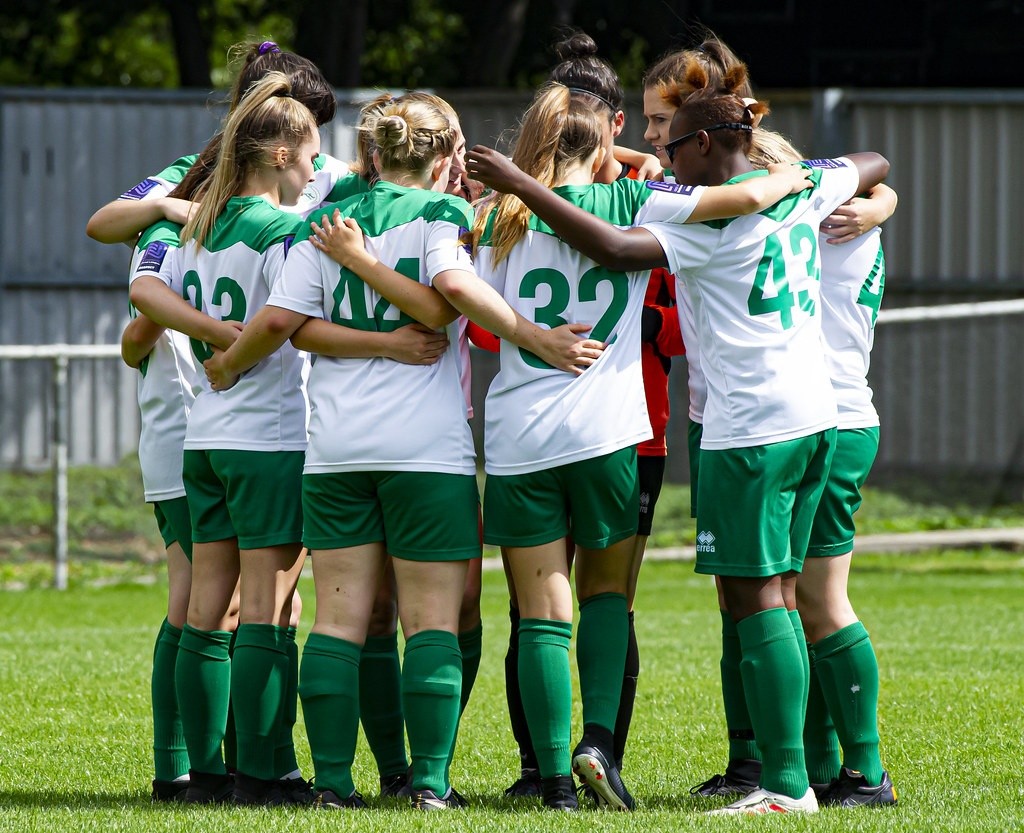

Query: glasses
[665,122,740,161]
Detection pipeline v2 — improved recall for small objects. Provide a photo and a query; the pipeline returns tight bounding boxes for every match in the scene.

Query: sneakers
[702,784,821,814]
[541,778,579,808]
[151,779,190,797]
[694,759,763,800]
[234,773,287,802]
[315,788,370,803]
[576,737,637,813]
[382,773,408,803]
[503,772,541,800]
[404,782,465,811]
[184,769,232,799]
[280,776,314,803]
[821,766,896,807]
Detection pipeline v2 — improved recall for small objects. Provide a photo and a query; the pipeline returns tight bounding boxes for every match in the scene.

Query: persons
[120,70,451,805]
[307,82,814,799]
[123,131,248,800]
[319,91,664,798]
[469,34,684,795]
[746,125,907,808]
[644,32,765,794]
[202,88,604,807]
[85,37,359,245]
[461,62,888,818]
